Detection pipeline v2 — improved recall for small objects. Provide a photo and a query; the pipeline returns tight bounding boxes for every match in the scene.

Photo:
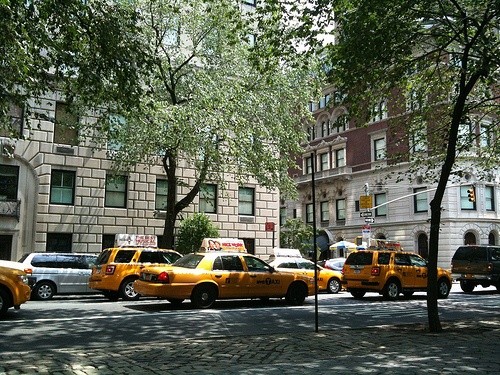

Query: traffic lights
[467,187,476,203]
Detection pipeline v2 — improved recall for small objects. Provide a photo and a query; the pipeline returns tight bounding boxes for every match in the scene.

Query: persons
[215,241,222,250]
[208,240,216,250]
[377,241,387,250]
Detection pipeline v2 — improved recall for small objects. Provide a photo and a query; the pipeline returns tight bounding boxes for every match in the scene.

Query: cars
[317,257,347,274]
[133,237,319,310]
[253,247,343,294]
[0,259,32,321]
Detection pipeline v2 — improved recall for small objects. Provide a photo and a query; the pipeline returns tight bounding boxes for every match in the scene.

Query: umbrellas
[330,240,356,257]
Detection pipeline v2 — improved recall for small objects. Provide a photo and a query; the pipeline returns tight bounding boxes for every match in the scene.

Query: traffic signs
[360,211,372,218]
[364,217,375,223]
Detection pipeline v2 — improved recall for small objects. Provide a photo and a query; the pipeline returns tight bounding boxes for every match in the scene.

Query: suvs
[16,251,102,301]
[450,243,500,294]
[341,238,454,302]
[88,233,195,301]
